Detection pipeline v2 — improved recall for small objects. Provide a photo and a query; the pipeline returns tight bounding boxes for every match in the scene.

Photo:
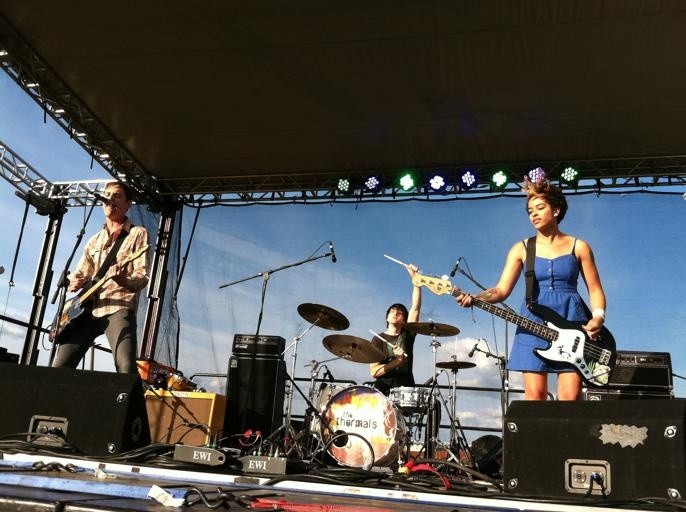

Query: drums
[320,386,406,469]
[309,384,347,439]
[167,371,197,392]
[387,387,432,415]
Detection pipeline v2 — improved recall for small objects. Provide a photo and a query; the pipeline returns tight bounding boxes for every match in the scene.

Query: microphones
[325,366,334,383]
[468,339,480,358]
[450,258,461,277]
[93,193,111,206]
[329,242,336,262]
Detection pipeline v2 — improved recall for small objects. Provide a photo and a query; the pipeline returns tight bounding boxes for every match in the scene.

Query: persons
[369,261,443,459]
[455,184,606,402]
[50,181,153,450]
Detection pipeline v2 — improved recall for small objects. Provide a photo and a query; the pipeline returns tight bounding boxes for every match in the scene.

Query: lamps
[335,162,583,195]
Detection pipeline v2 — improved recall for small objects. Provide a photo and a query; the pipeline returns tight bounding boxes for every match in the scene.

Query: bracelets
[592,308,606,319]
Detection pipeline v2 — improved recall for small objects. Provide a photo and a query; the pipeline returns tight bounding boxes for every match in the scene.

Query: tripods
[413,333,473,482]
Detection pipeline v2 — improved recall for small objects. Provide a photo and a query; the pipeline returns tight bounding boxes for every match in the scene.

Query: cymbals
[298,303,350,330]
[435,362,475,369]
[405,323,459,336]
[322,334,384,363]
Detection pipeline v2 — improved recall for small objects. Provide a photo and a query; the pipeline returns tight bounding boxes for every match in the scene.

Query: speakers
[472,435,502,475]
[145,389,226,447]
[0,361,151,464]
[582,350,673,390]
[504,398,686,510]
[581,387,674,401]
[221,356,286,456]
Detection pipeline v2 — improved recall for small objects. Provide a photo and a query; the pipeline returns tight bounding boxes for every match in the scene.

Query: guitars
[412,274,617,389]
[49,243,151,342]
